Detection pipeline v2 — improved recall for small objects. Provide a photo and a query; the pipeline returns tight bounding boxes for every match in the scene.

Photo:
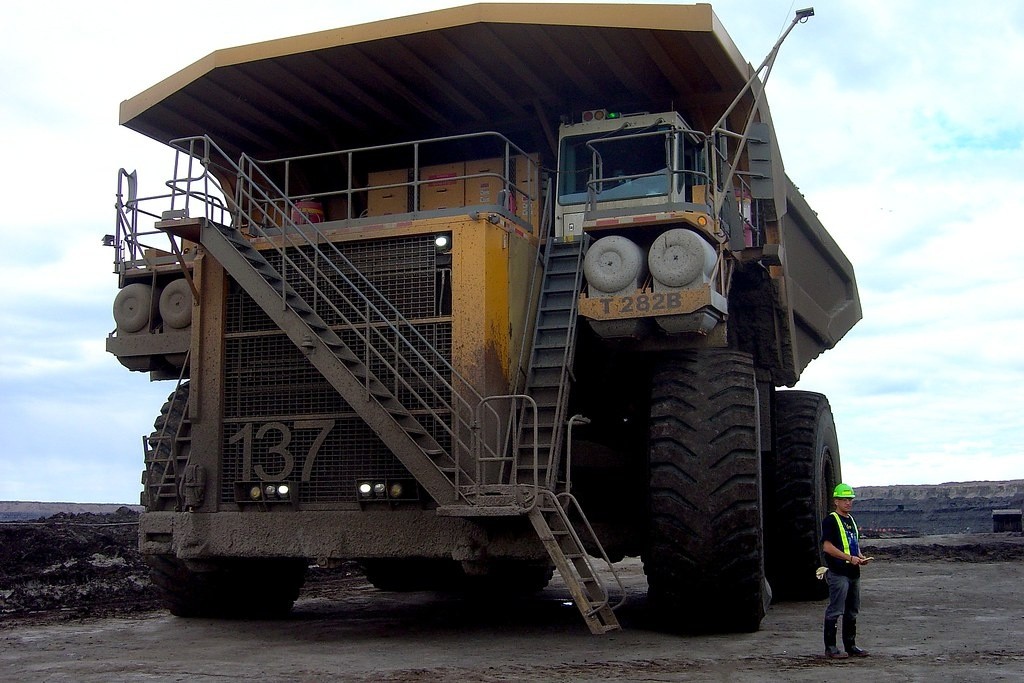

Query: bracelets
[850,555,853,563]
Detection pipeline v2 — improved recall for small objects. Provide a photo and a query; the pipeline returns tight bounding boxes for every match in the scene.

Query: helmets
[832,483,856,498]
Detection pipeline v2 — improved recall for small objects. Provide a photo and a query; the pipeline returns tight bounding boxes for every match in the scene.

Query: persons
[822,483,874,659]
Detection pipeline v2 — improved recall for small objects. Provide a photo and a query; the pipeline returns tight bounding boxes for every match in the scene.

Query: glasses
[839,498,855,502]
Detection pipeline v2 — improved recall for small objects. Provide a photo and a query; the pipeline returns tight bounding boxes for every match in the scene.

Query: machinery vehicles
[101,3,864,636]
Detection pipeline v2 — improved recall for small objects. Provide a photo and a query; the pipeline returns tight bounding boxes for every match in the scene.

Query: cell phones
[862,557,873,563]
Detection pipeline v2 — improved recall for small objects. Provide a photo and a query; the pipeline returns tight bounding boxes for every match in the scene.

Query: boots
[841,622,870,657]
[824,625,849,658]
